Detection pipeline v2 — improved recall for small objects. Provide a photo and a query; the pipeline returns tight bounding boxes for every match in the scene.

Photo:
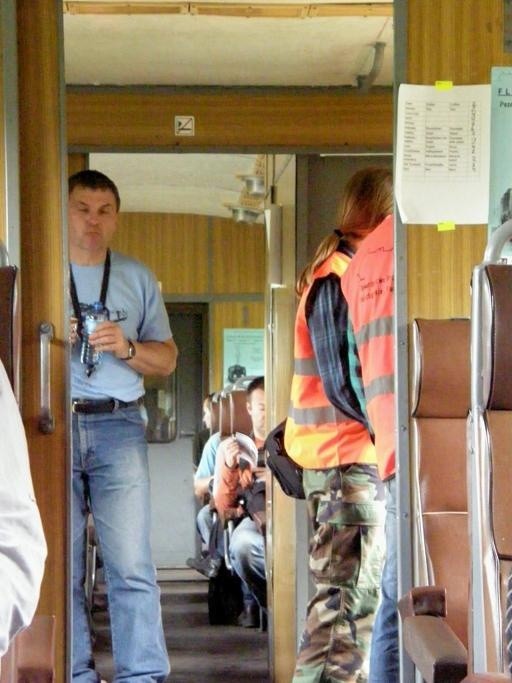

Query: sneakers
[185,553,221,581]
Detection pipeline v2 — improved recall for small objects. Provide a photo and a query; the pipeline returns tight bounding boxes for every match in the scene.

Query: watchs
[116,337,137,365]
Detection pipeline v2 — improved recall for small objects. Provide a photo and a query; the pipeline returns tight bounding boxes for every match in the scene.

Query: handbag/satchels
[262,413,307,501]
[206,555,247,628]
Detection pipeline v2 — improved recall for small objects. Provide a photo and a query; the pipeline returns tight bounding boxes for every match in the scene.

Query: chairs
[209,375,264,576]
[466,220,512,683]
[395,317,472,683]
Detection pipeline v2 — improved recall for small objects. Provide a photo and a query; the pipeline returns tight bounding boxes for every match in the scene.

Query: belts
[71,397,141,415]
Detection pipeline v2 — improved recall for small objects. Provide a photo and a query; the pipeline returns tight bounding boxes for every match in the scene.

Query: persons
[183,372,273,630]
[66,165,179,683]
[276,162,393,683]
[1,347,48,661]
[340,206,406,683]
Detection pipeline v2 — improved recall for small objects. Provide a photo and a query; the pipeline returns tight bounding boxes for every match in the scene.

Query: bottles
[79,300,105,364]
